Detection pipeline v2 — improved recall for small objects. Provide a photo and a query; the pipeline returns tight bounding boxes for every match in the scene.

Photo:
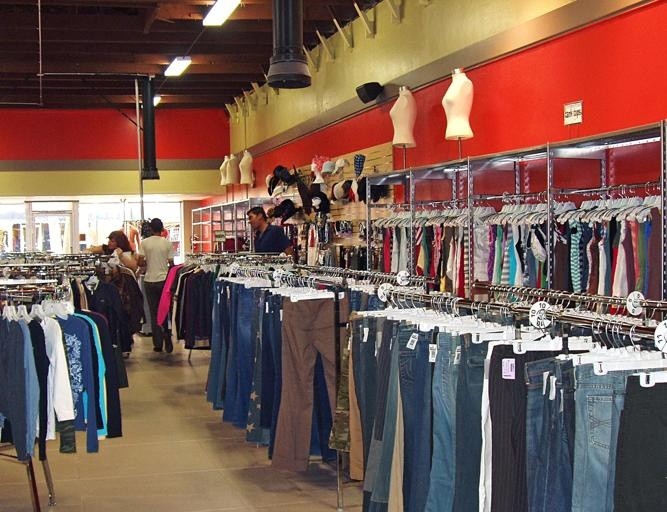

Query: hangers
[1,287,72,323]
[234,264,383,288]
[373,183,664,230]
[382,268,664,355]
[2,252,121,286]
[182,254,289,273]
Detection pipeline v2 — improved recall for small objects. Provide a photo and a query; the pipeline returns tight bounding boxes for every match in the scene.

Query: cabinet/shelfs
[190,196,268,253]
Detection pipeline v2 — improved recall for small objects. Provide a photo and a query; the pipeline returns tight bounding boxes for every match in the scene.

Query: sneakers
[152,347,163,352]
[163,334,174,353]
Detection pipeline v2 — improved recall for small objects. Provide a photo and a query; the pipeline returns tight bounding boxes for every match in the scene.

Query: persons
[441,67,474,141]
[238,149,254,184]
[106,231,138,274]
[137,218,175,353]
[219,155,230,186]
[133,221,173,337]
[389,84,418,149]
[246,206,294,256]
[310,192,327,211]
[225,153,238,185]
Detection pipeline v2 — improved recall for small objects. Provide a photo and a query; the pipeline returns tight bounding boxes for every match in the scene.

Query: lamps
[202,0,243,29]
[164,57,193,80]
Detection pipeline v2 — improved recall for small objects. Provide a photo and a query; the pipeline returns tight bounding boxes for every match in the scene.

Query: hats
[263,174,279,197]
[331,158,350,176]
[265,207,275,219]
[280,198,296,213]
[272,165,288,181]
[272,185,284,200]
[272,205,283,218]
[329,176,382,204]
[320,160,335,175]
[285,175,297,186]
